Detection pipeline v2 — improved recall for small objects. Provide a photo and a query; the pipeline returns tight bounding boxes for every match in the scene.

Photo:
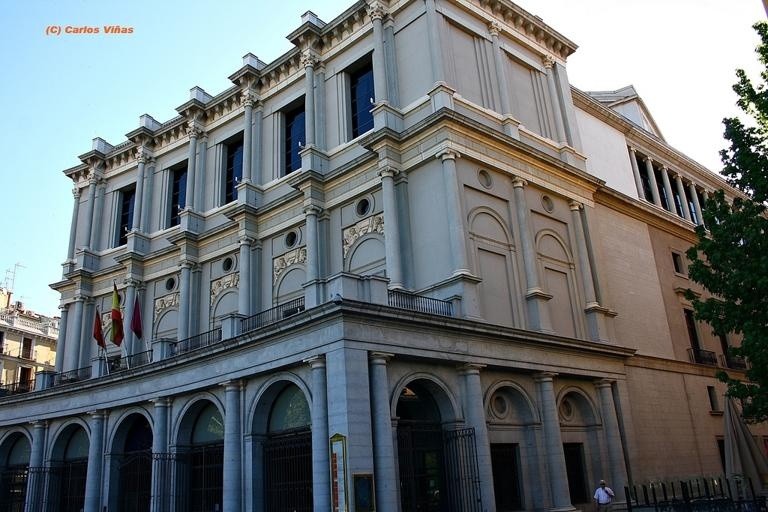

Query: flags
[93,308,106,349]
[109,282,124,347]
[130,294,142,340]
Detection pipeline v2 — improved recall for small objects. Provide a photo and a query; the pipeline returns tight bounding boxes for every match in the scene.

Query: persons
[593,479,615,512]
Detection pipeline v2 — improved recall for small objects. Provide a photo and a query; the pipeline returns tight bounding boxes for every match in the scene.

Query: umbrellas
[723,390,767,501]
